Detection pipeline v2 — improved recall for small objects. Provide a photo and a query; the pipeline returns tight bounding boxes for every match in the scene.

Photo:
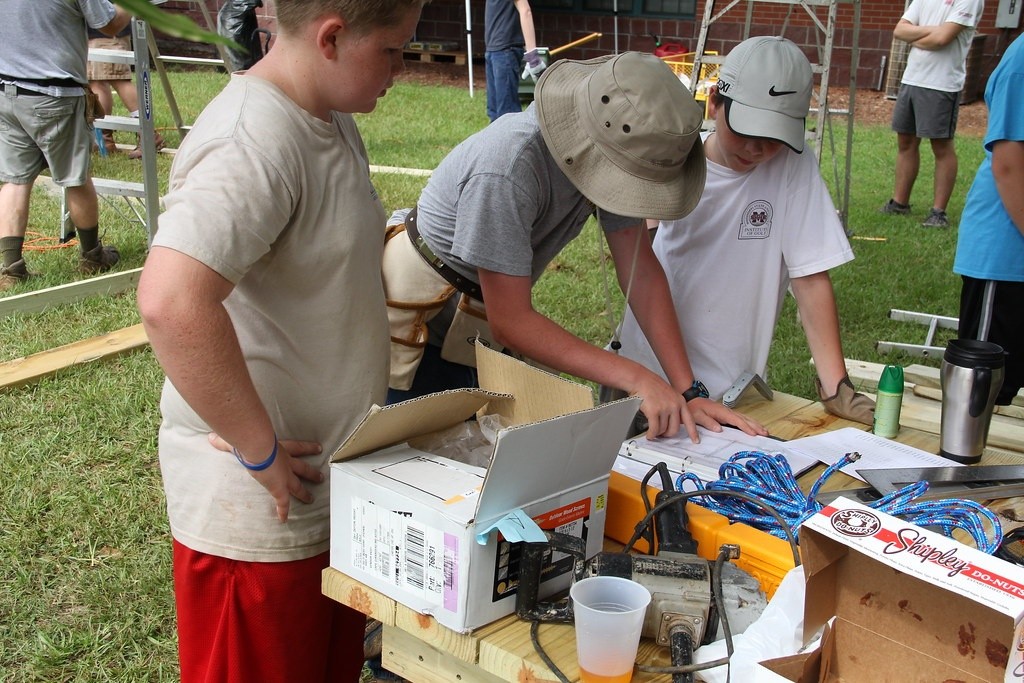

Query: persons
[599,36,901,442]
[875,0,985,228]
[363,50,769,683]
[485,0,547,124]
[953,28,1024,407]
[137,0,428,683]
[0,0,120,292]
[87,0,167,161]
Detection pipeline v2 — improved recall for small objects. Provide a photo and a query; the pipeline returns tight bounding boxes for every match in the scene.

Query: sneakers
[878,199,911,216]
[78,238,120,278]
[0,258,40,292]
[923,208,948,228]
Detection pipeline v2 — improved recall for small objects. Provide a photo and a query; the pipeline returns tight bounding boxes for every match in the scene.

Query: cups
[569,576,652,683]
[939,339,1005,464]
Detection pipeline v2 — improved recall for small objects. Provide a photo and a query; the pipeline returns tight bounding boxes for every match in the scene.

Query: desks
[321,389,1024,683]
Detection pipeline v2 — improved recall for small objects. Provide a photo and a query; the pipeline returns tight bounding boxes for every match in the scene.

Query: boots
[128,117,166,159]
[90,128,117,154]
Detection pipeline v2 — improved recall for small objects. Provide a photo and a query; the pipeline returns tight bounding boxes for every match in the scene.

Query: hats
[718,35,813,154]
[534,51,706,220]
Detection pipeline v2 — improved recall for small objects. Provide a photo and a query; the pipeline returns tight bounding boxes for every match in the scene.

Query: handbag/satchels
[441,292,504,368]
[381,224,457,391]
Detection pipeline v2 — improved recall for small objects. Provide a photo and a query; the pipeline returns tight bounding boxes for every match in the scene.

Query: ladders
[57,15,192,253]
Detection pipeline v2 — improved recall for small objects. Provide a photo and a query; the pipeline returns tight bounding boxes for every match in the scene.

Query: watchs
[681,380,710,403]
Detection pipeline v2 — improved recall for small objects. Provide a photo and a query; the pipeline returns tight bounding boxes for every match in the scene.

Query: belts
[0,83,49,96]
[406,205,485,303]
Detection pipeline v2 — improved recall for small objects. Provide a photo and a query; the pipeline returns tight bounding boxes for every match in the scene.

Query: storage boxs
[330,339,643,634]
[755,496,1024,682]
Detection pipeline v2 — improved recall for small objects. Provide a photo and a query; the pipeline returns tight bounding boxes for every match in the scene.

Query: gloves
[815,372,900,431]
[521,48,546,84]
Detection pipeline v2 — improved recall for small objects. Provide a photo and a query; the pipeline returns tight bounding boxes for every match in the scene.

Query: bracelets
[234,431,278,471]
